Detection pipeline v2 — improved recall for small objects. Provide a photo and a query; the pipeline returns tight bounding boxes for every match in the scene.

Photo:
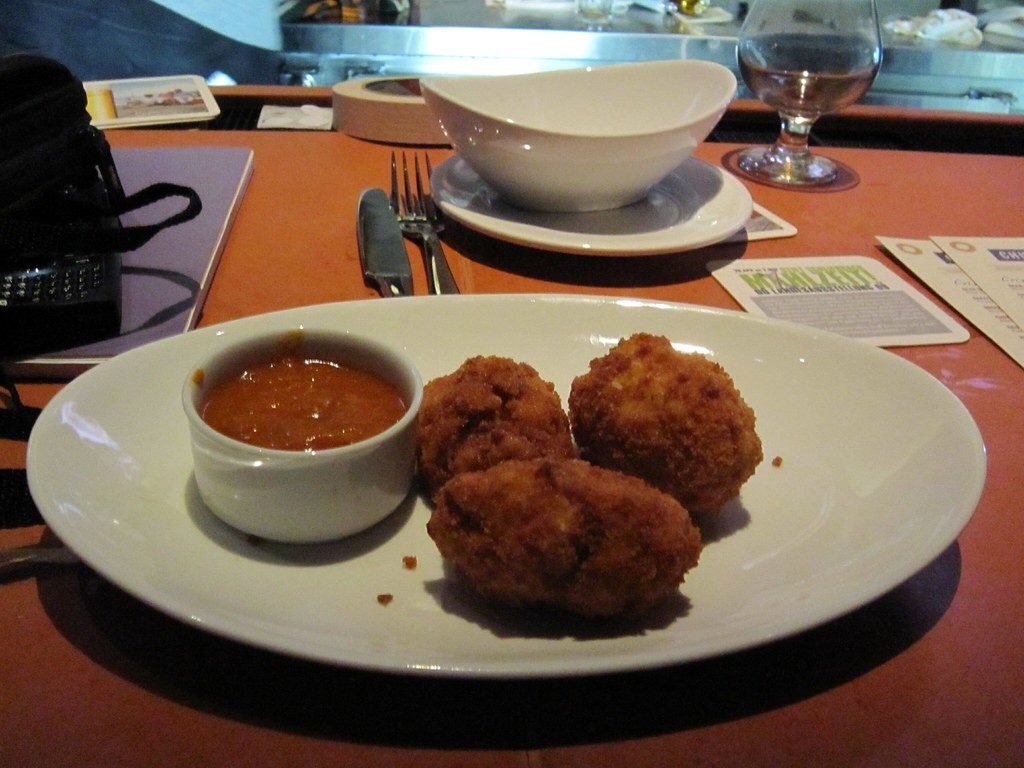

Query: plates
[433,151,762,257]
[26,285,990,674]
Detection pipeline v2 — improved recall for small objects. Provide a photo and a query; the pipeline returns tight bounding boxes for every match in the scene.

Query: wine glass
[723,0,882,190]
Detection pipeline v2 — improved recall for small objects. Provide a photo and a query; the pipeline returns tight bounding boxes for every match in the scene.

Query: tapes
[332,76,452,144]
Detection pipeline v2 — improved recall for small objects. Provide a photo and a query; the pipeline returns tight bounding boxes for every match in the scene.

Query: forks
[384,150,462,299]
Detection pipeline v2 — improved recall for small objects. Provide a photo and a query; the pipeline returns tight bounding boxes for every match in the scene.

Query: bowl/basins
[181,331,421,545]
[417,60,738,216]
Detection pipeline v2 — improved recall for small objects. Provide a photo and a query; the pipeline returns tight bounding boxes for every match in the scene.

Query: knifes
[352,185,420,297]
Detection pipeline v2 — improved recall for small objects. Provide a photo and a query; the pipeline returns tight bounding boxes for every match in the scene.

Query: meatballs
[426,456,706,622]
[567,331,770,519]
[411,354,576,502]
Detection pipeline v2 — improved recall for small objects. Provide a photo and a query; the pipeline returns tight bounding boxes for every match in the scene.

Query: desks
[0,83,1024,768]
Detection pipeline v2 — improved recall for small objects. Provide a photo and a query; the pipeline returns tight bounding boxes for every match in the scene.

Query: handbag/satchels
[0,54,202,257]
